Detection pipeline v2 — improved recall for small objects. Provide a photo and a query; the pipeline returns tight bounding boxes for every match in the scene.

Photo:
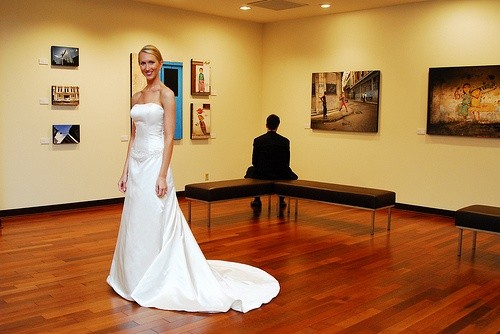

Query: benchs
[184,178,396,236]
[454,205,500,256]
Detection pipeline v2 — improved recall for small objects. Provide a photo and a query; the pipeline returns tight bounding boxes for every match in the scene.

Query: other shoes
[280,201,287,208]
[250,200,262,207]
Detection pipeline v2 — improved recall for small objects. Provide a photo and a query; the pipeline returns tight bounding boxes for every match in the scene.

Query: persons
[105,44,281,314]
[339,94,348,112]
[244,114,298,209]
[320,92,328,117]
